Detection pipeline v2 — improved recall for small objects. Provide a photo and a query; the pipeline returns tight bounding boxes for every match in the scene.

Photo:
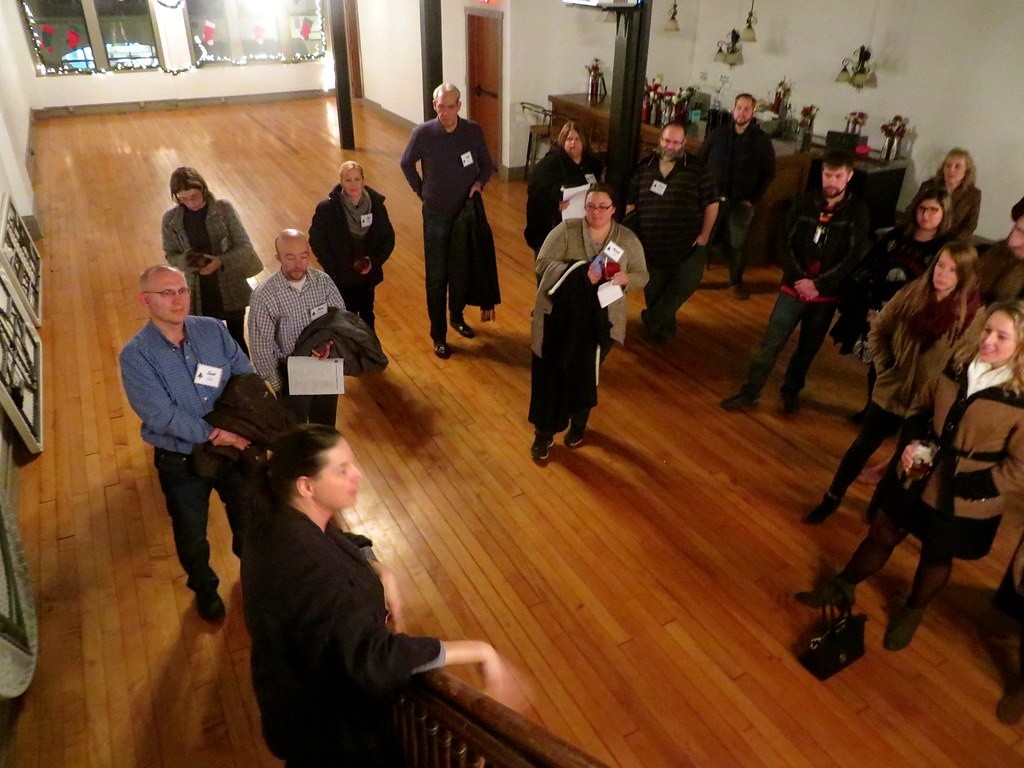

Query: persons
[792,146,1024,723]
[722,149,868,417]
[625,121,719,345]
[248,229,347,429]
[524,121,607,288]
[161,167,263,360]
[119,266,258,622]
[401,83,492,357]
[239,423,504,768]
[309,160,395,330]
[529,183,651,460]
[696,94,776,296]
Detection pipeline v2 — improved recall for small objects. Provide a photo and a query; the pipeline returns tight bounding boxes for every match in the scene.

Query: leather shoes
[450,319,475,339]
[434,336,447,358]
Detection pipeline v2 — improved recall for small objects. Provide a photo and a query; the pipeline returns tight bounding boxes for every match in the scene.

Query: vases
[670,106,679,122]
[795,130,813,153]
[641,96,650,122]
[879,136,900,163]
[588,75,599,95]
[663,106,672,126]
[661,108,667,125]
[650,102,657,124]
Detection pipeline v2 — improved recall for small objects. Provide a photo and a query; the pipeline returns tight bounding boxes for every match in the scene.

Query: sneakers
[530,431,554,459]
[565,426,588,446]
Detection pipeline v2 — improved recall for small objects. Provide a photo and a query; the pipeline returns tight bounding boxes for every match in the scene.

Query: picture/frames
[0,265,43,455]
[0,191,43,329]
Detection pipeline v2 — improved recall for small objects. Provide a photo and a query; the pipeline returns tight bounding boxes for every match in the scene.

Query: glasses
[583,202,614,213]
[661,135,685,145]
[144,287,190,297]
[179,188,203,203]
[919,205,945,214]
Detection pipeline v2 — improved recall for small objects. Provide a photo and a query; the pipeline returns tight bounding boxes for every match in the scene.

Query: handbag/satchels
[800,592,868,681]
[217,201,264,279]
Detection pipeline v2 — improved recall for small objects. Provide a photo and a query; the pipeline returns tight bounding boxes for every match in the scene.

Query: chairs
[520,101,581,183]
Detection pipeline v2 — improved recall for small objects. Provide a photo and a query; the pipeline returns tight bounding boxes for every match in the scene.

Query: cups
[908,437,934,480]
[603,256,620,279]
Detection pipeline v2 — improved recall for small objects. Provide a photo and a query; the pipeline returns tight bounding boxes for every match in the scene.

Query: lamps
[837,46,874,90]
[712,28,741,67]
[663,0,681,33]
[739,0,758,44]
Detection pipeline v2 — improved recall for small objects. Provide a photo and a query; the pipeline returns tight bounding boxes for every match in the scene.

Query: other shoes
[884,603,928,651]
[806,493,842,524]
[728,276,751,301]
[795,573,857,612]
[721,390,760,411]
[641,308,676,348]
[195,590,226,623]
[783,386,801,414]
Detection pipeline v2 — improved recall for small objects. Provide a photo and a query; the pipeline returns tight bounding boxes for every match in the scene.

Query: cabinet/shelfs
[807,134,908,241]
[548,93,813,268]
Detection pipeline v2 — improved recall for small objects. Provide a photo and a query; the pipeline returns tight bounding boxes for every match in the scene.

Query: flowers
[880,115,908,140]
[844,111,868,126]
[798,104,820,131]
[641,73,699,105]
[584,59,603,79]
